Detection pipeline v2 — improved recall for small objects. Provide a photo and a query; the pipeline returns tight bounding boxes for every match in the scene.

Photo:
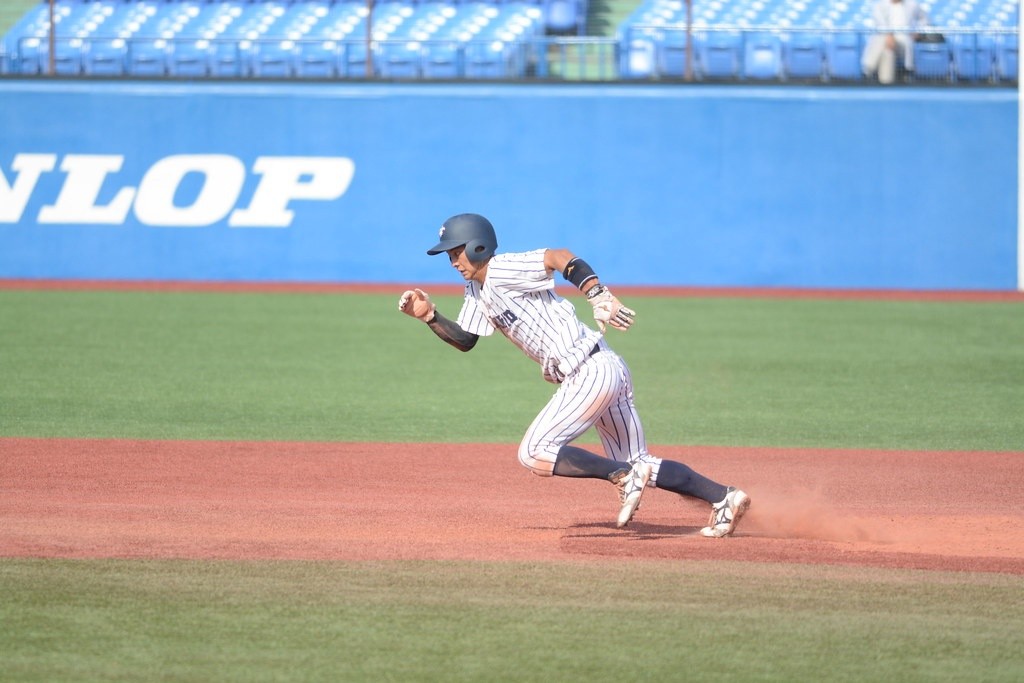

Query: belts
[588,343,601,356]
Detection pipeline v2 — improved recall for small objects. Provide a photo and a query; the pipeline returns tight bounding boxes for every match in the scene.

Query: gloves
[398,288,436,322]
[587,286,636,334]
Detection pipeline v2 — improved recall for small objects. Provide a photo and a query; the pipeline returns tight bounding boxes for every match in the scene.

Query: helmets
[427,213,498,263]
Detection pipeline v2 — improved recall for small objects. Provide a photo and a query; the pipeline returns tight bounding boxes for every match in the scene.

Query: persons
[861,0,929,84]
[542,0,589,52]
[398,214,751,537]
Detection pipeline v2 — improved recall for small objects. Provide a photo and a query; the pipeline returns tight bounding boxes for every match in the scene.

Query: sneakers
[700,485,750,538]
[608,460,652,528]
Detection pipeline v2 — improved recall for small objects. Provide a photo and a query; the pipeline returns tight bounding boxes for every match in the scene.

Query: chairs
[612,0,1020,88]
[0,0,588,88]
